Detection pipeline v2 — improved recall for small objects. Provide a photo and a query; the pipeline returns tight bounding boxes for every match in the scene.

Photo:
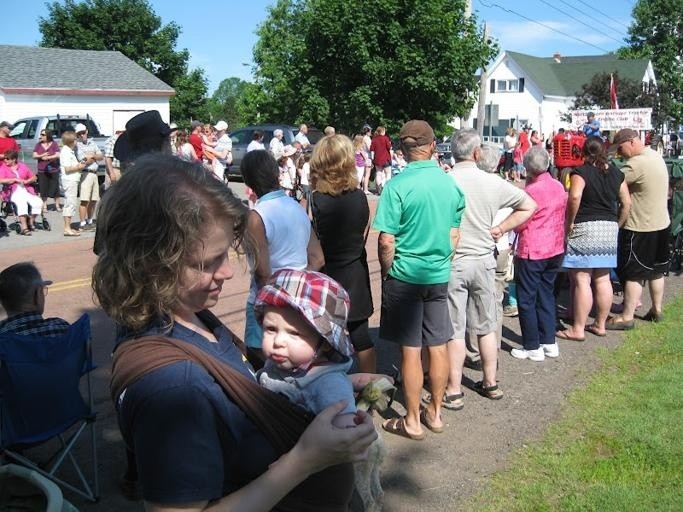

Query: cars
[219,123,326,179]
[670,130,683,149]
[497,148,511,177]
[436,142,452,156]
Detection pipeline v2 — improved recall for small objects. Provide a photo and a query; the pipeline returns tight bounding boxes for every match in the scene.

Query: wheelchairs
[0,183,50,235]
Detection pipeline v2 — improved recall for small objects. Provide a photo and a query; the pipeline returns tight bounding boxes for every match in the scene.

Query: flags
[610,74,618,110]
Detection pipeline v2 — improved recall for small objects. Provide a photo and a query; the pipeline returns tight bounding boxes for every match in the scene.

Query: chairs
[0,314,101,511]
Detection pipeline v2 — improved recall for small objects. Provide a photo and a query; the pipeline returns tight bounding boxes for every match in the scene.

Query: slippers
[382,417,426,440]
[555,329,585,341]
[586,322,606,336]
[421,409,444,433]
[64,229,80,236]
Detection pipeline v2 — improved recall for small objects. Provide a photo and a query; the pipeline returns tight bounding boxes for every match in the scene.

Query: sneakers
[78,221,97,232]
[510,342,544,361]
[645,309,664,322]
[503,305,519,317]
[606,316,635,330]
[541,343,560,357]
[610,297,643,312]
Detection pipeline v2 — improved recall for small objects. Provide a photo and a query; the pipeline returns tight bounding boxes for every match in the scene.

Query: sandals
[472,381,504,400]
[20,221,37,236]
[422,390,464,410]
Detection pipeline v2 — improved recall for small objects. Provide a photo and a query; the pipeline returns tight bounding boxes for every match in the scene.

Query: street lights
[241,61,261,122]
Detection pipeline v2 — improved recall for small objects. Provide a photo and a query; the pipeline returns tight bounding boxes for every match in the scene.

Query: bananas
[356,377,396,411]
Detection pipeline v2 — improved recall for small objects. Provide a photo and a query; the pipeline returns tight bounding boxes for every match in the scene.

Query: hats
[0,122,14,129]
[75,124,86,133]
[253,269,355,357]
[113,111,181,162]
[282,144,297,157]
[399,120,435,147]
[191,120,204,129]
[213,121,228,131]
[608,128,638,145]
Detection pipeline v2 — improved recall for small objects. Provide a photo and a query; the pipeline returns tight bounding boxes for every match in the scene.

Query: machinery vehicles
[544,128,667,190]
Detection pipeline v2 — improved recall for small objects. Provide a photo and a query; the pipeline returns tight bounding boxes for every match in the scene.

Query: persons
[501,111,672,363]
[371,120,538,439]
[251,269,357,429]
[301,133,378,377]
[1,261,71,338]
[240,150,326,373]
[96,148,395,510]
[247,122,408,216]
[114,110,233,182]
[1,120,128,236]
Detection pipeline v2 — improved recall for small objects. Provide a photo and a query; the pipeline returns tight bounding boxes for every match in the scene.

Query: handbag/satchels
[496,247,515,281]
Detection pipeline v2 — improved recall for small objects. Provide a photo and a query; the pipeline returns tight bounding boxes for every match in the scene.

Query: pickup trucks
[5,113,112,191]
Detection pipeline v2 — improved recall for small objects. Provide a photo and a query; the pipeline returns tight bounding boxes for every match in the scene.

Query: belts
[82,169,97,173]
